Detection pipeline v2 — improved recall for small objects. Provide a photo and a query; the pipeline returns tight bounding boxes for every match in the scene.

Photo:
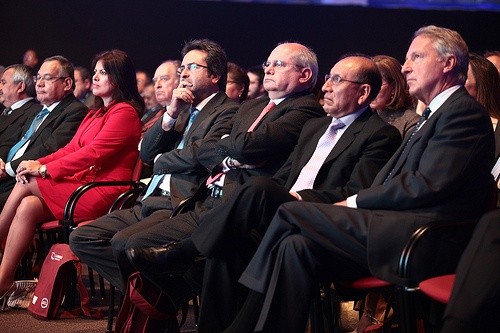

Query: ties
[6,109,49,163]
[206,101,276,187]
[140,108,165,140]
[384,108,430,182]
[141,110,199,201]
[290,119,346,193]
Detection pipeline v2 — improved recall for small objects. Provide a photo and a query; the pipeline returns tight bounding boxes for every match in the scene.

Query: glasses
[33,75,69,83]
[176,64,208,75]
[261,61,304,69]
[325,74,366,86]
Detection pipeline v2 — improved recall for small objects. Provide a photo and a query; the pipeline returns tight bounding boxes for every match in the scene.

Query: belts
[158,190,171,197]
[208,186,222,198]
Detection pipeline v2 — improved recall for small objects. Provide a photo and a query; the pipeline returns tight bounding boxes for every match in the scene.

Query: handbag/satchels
[30,243,90,320]
[7,277,39,309]
[115,273,164,333]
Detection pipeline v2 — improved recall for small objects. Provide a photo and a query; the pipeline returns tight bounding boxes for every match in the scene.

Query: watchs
[41,165,46,178]
[230,158,236,166]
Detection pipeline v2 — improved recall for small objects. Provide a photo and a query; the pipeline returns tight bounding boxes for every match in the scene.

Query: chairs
[24,155,500,333]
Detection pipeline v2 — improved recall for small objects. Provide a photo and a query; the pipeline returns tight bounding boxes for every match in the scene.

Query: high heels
[0,280,17,310]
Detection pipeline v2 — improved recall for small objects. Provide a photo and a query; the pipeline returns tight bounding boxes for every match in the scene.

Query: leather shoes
[126,242,191,277]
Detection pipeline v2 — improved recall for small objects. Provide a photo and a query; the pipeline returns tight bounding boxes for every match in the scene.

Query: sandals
[351,306,393,333]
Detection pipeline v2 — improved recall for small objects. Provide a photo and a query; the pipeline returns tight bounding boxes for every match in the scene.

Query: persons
[0,26,500,333]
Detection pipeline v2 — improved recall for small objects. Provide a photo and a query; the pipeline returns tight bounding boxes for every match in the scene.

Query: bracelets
[38,166,41,176]
